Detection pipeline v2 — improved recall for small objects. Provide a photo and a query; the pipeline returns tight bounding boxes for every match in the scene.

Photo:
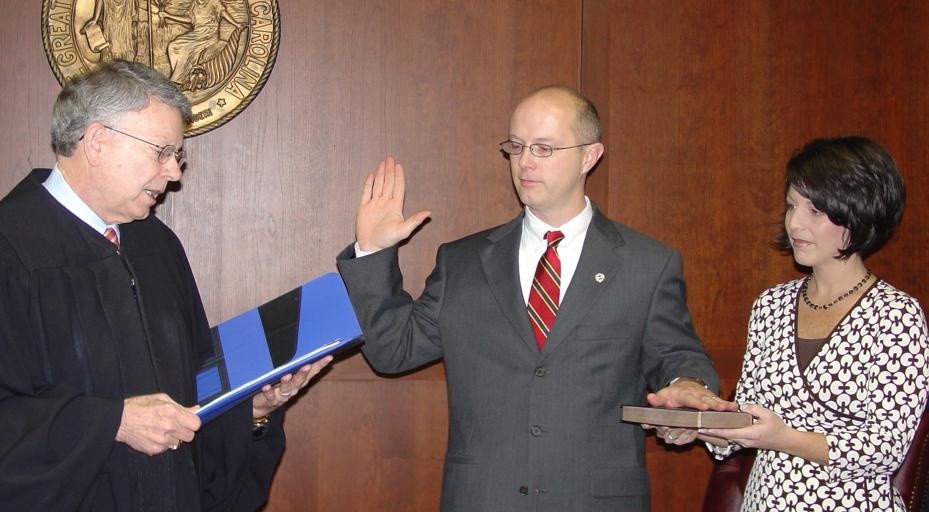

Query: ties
[525,228,565,353]
[102,227,121,256]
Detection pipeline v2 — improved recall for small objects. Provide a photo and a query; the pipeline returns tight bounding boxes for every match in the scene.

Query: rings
[667,433,673,440]
[711,394,718,397]
[727,439,733,444]
[170,441,180,451]
[278,391,292,396]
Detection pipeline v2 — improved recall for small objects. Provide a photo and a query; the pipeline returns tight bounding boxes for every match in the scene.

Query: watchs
[253,416,270,431]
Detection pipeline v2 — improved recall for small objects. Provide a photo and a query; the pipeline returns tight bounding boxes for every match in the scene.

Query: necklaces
[801,267,873,311]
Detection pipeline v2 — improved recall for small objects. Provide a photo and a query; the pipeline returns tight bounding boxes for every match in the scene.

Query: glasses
[498,141,593,158]
[78,124,187,170]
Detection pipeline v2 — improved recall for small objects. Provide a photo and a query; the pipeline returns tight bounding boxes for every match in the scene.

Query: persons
[157,0,247,86]
[638,133,927,511]
[0,58,335,510]
[79,0,147,66]
[336,85,740,512]
